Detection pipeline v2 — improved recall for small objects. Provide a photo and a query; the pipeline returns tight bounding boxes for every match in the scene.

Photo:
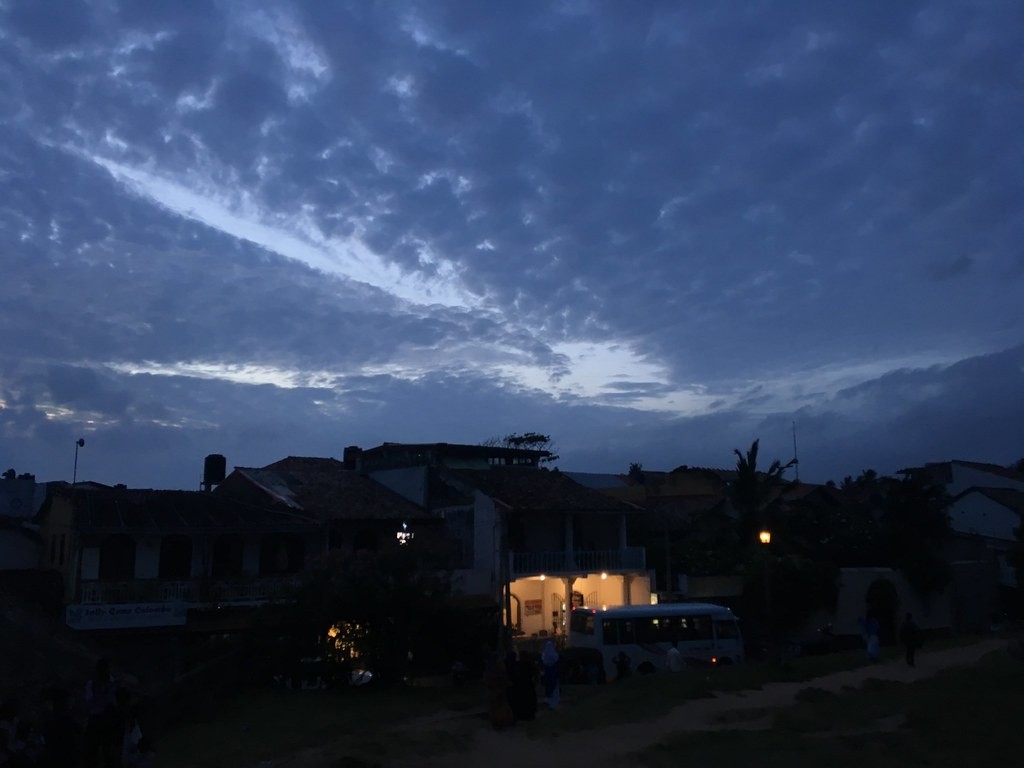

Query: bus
[570,603,747,682]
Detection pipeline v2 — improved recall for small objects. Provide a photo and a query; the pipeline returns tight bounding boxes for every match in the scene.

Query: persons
[85,655,120,768]
[505,646,537,722]
[859,613,880,656]
[541,640,564,710]
[0,697,48,768]
[899,612,923,668]
